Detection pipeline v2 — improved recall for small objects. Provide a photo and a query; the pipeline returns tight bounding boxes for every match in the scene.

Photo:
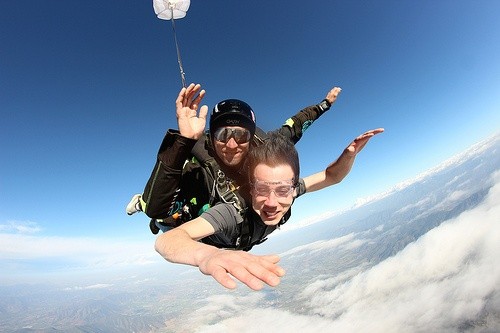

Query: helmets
[209,99,256,140]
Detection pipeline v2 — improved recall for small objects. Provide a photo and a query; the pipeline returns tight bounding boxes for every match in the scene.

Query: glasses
[253,177,294,198]
[214,127,250,144]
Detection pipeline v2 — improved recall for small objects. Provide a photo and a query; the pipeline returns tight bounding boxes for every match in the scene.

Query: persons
[155,128,385,292]
[126,83,342,227]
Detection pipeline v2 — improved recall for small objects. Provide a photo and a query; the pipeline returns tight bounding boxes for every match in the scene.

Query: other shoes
[126,194,141,215]
[149,219,160,234]
